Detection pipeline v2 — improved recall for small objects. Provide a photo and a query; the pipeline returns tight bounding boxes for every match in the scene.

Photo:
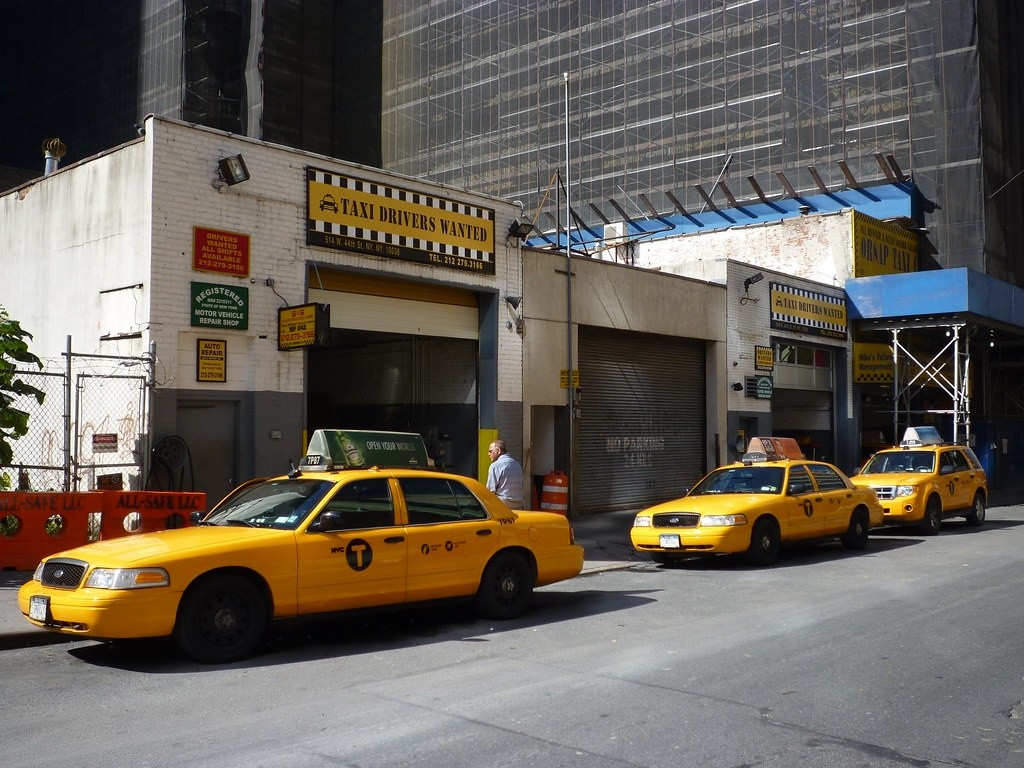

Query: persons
[485,439,524,511]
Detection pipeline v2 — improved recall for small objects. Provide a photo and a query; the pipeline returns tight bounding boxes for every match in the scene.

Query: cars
[849,426,990,536]
[16,429,586,666]
[630,437,884,568]
